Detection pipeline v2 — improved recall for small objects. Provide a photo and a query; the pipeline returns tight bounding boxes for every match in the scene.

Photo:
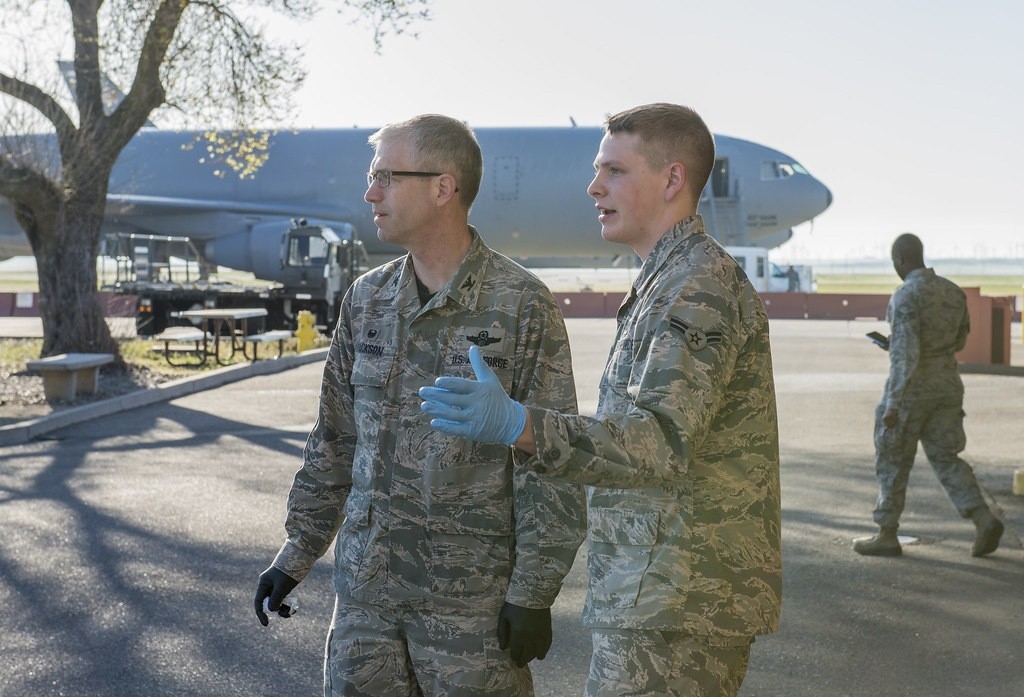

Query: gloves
[254,566,297,626]
[418,346,526,446]
[498,602,553,668]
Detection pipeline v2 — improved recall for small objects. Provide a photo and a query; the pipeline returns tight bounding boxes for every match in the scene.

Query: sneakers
[971,514,1004,557]
[853,528,903,556]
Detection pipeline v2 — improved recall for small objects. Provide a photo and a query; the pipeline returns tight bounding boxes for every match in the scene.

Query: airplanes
[0,126,833,293]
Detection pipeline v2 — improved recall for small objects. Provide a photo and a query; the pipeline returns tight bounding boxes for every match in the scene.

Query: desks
[179,307,269,367]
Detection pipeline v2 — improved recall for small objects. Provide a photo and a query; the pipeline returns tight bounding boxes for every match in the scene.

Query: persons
[415,98,784,697]
[851,231,1003,560]
[255,112,589,697]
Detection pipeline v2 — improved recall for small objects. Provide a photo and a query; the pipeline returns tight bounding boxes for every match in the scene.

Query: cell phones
[867,332,890,348]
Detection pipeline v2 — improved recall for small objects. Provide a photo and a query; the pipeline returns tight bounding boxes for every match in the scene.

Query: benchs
[27,350,115,402]
[157,331,213,367]
[240,328,293,363]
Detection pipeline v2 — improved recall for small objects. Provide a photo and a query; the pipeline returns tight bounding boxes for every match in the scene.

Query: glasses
[366,171,460,194]
